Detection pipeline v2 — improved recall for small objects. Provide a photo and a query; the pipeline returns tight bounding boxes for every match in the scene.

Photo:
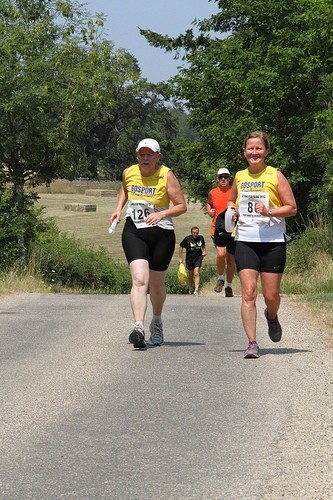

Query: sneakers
[264,308,282,342]
[128,324,146,348]
[213,279,224,292]
[243,341,260,358]
[149,321,164,345]
[225,286,233,297]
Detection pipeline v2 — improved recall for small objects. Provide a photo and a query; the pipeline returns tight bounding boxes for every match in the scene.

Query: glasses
[218,175,229,179]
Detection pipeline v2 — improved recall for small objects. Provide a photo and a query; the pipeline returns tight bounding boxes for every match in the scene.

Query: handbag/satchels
[178,262,188,284]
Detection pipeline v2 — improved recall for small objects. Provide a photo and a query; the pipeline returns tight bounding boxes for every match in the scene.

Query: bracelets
[268,209,272,216]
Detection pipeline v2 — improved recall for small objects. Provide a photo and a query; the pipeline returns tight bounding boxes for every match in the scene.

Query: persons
[110,138,187,348]
[179,227,207,295]
[228,131,297,358]
[206,168,236,297]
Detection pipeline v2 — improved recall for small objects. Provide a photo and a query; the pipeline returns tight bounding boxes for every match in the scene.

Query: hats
[217,168,230,175]
[135,138,161,154]
